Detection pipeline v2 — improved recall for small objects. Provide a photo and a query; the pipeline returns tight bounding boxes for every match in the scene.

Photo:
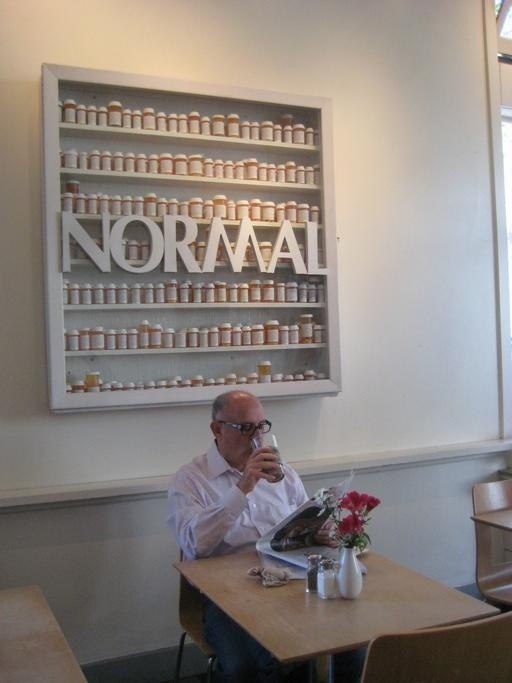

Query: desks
[470,506,512,533]
[172,550,501,683]
[0,584,87,683]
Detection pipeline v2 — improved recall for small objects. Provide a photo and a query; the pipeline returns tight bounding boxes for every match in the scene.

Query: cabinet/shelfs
[42,64,342,413]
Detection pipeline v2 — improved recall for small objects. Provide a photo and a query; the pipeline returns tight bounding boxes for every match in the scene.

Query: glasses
[218,420,272,437]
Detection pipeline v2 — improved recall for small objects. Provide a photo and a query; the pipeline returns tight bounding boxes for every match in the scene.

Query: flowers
[316,488,381,549]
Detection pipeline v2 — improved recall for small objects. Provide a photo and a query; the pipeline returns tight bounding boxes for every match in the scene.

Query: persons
[169,389,366,683]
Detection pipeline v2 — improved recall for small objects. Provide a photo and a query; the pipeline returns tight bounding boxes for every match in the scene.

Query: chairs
[471,479,512,606]
[174,547,217,682]
[360,610,512,683]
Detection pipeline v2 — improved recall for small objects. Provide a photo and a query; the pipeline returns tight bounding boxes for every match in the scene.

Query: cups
[250,432,285,483]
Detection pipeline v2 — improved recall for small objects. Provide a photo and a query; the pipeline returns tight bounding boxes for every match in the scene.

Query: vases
[337,549,364,601]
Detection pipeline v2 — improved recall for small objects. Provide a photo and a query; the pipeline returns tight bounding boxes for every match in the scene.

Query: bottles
[62,180,321,224]
[63,279,325,304]
[64,313,328,352]
[69,237,324,265]
[60,98,321,147]
[62,148,322,188]
[304,554,338,600]
[65,360,328,394]
